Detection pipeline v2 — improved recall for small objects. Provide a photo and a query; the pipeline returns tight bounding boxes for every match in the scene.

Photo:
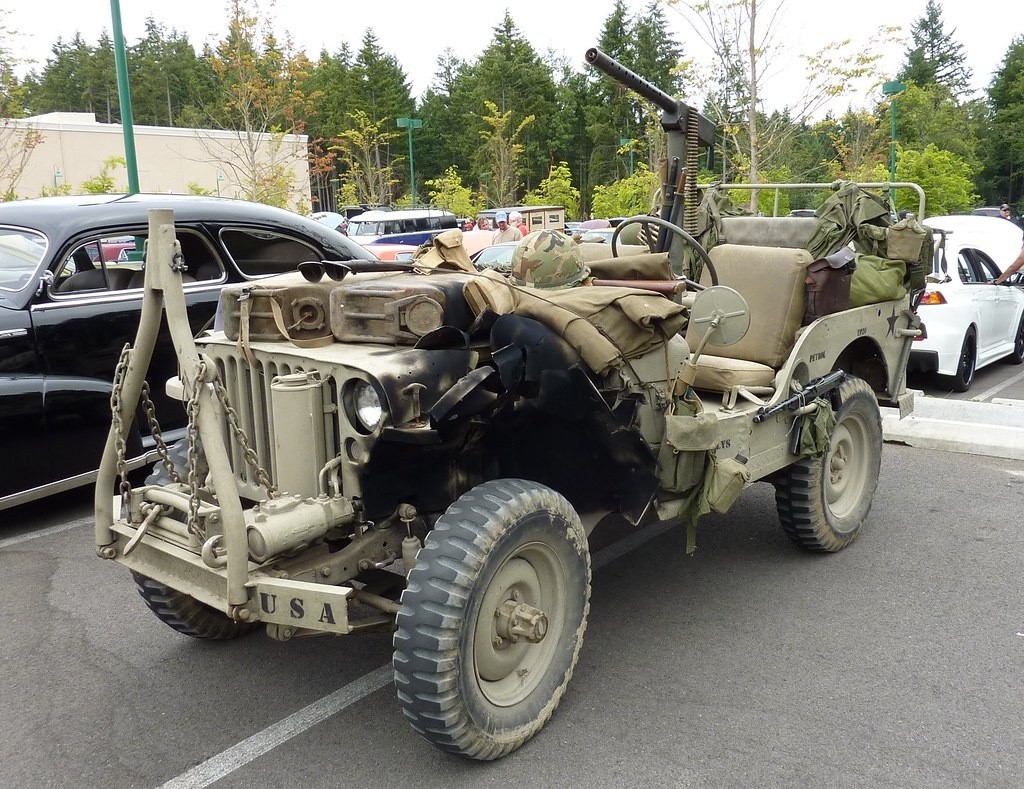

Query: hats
[1000,204,1011,210]
[495,211,507,222]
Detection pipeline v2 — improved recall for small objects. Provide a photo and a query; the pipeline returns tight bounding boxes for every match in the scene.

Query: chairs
[58,268,134,293]
[127,271,197,289]
[686,243,814,404]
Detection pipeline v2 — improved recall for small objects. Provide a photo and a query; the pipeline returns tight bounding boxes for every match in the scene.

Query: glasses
[1002,209,1009,212]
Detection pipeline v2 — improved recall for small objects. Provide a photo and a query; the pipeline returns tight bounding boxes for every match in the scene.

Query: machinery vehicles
[91,46,950,765]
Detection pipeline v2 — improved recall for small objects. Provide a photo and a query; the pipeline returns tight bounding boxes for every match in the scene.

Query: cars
[0,192,386,522]
[908,206,1024,393]
[300,204,640,277]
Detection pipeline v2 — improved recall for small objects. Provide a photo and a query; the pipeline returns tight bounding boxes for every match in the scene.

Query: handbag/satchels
[463,268,520,320]
[433,228,477,274]
[653,375,721,493]
[805,246,856,324]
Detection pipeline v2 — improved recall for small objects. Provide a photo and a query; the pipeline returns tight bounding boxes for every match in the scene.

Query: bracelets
[992,278,998,285]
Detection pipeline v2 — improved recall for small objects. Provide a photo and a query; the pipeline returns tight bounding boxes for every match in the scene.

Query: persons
[988,245,1024,285]
[994,203,1021,227]
[464,210,528,245]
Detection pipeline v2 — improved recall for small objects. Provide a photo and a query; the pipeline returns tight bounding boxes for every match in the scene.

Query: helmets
[508,229,591,291]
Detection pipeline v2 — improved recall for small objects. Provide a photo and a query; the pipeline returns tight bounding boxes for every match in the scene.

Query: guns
[498,273,687,295]
[752,369,847,456]
